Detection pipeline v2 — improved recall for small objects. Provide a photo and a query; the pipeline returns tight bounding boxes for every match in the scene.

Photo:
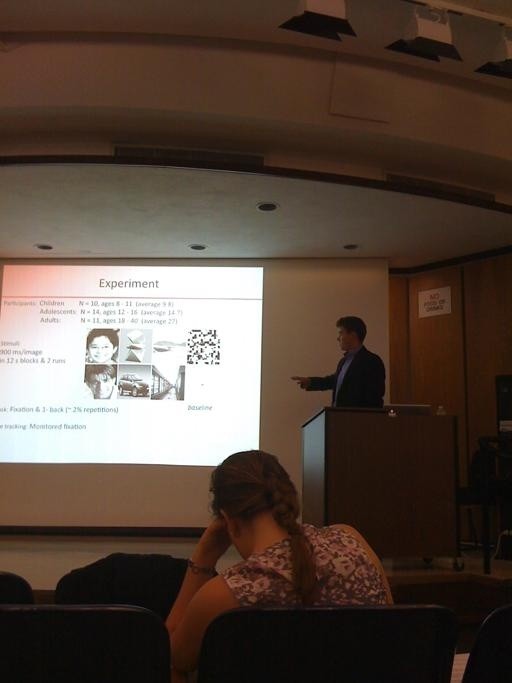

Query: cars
[117,372,150,398]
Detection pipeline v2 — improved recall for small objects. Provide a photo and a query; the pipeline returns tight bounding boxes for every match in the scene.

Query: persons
[289,315,385,409]
[85,328,118,363]
[159,447,405,683]
[84,365,117,399]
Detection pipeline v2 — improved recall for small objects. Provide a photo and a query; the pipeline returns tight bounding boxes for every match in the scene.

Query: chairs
[2,604,174,680]
[197,606,457,681]
[461,604,512,683]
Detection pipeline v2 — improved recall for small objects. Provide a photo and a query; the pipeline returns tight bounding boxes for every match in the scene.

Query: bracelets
[184,557,218,576]
